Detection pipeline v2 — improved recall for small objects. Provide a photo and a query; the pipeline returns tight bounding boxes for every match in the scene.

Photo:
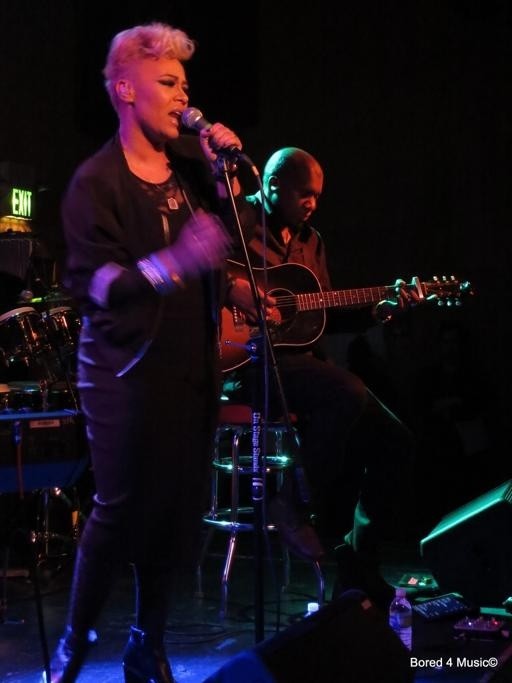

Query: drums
[0,307,54,379]
[0,379,81,415]
[41,307,83,368]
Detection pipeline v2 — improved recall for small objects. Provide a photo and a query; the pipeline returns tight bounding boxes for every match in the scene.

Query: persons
[221,140,424,567]
[35,19,246,683]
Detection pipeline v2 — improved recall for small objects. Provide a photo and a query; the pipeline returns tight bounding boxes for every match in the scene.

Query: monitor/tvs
[182,106,242,158]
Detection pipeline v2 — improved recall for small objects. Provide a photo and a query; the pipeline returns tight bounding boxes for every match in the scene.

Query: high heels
[121,625,174,683]
[43,627,87,683]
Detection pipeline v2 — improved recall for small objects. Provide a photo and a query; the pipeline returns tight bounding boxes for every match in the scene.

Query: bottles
[388,589,412,651]
[302,603,318,618]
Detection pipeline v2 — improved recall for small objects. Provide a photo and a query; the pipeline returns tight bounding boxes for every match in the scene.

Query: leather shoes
[273,513,323,562]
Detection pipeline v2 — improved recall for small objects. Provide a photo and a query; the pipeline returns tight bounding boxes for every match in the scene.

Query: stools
[191,404,325,618]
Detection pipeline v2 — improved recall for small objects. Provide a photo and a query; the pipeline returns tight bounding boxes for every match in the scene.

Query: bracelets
[134,249,185,292]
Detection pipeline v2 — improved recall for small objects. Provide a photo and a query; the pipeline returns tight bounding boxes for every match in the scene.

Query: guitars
[219,260,474,373]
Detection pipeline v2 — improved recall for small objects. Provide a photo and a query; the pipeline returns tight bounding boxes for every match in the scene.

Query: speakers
[419,479,512,606]
[200,589,417,683]
